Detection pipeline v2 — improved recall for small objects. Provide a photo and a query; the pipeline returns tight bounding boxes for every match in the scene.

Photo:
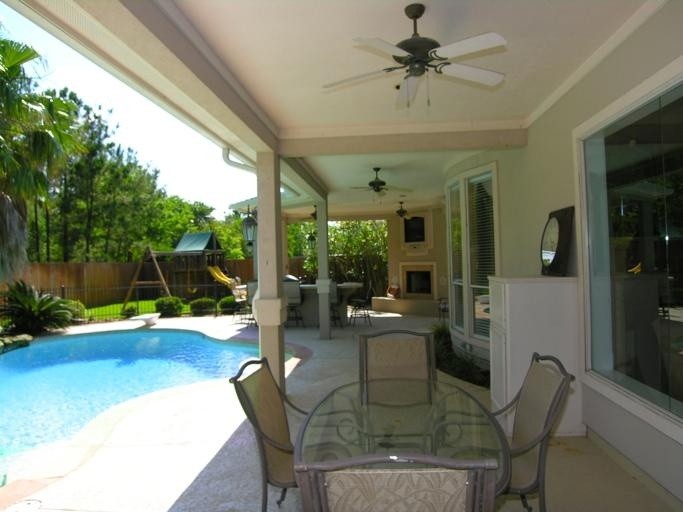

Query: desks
[294,378,508,497]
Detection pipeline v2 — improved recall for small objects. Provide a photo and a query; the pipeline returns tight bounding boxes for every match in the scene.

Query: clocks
[538,206,575,275]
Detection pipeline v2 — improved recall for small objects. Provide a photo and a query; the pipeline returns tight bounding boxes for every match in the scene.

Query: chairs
[293,447,500,511]
[355,328,442,455]
[234,272,375,329]
[432,352,577,512]
[227,357,360,511]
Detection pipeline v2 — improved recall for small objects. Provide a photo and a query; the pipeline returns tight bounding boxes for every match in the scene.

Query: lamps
[306,226,315,249]
[238,204,257,250]
[409,61,426,76]
[395,199,408,218]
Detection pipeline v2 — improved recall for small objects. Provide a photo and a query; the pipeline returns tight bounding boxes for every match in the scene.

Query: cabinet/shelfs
[487,274,586,440]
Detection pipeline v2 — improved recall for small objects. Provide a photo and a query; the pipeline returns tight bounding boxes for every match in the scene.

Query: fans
[346,167,414,201]
[319,0,507,109]
